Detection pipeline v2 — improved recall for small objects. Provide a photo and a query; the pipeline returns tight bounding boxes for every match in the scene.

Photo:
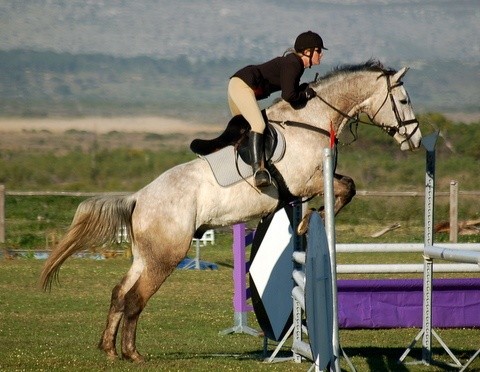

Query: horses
[38,54,425,366]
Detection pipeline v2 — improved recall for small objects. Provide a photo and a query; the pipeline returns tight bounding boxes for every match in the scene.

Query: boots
[248,130,272,188]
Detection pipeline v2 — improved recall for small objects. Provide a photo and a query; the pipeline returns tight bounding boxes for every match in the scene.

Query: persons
[227,31,328,187]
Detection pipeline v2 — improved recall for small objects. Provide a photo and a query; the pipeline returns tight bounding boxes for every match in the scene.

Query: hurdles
[216,128,480,372]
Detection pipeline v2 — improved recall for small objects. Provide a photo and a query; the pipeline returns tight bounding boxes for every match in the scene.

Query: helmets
[294,30,329,51]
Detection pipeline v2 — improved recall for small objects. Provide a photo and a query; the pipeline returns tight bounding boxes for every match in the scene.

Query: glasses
[315,49,322,54]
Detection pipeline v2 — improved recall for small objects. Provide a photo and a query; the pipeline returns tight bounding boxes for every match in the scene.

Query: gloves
[302,88,317,100]
[300,82,309,91]
